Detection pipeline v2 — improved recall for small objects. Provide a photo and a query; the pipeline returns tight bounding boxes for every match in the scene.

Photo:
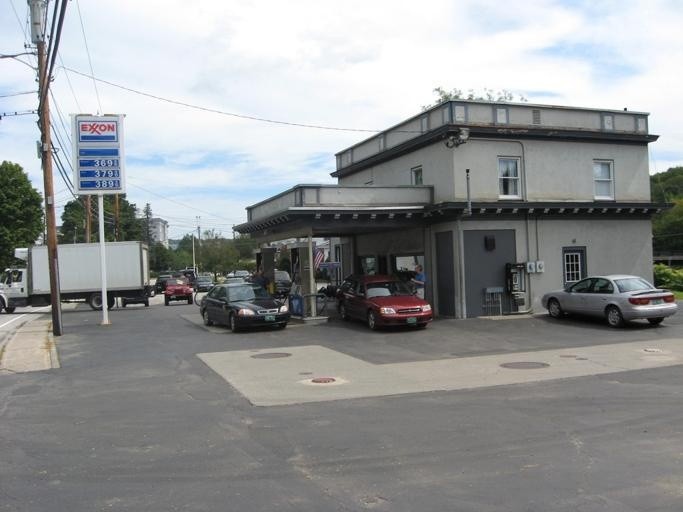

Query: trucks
[0,241,151,313]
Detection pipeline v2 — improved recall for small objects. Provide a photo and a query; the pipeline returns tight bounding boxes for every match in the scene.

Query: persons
[410,263,426,299]
[248,270,267,291]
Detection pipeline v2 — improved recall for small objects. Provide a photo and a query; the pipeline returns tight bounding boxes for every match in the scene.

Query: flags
[312,247,325,272]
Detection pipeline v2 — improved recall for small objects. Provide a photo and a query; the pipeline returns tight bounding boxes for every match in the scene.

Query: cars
[121,266,292,332]
[336,273,433,329]
[542,274,679,328]
